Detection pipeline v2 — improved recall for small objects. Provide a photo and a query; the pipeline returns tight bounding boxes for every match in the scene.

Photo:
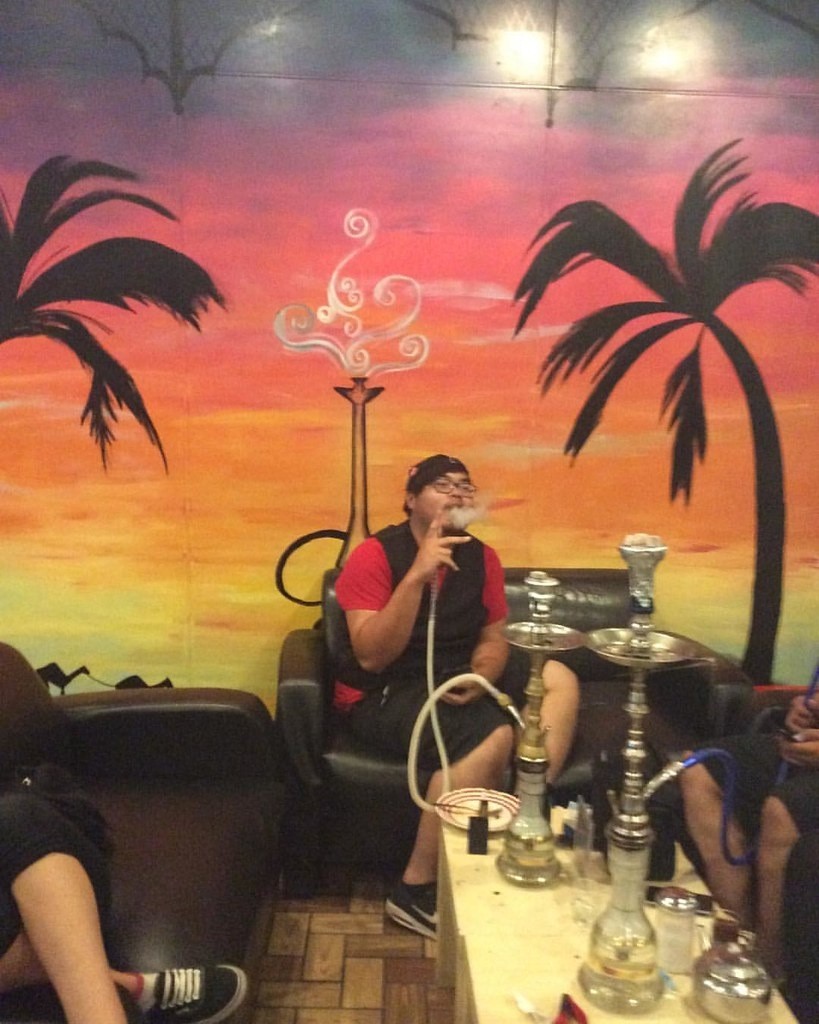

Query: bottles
[652,885,702,976]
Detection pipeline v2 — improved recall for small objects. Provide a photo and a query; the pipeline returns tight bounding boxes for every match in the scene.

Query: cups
[712,909,742,950]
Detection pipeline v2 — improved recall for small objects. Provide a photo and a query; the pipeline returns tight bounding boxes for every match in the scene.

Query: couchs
[0,641,284,1023]
[271,564,735,895]
[710,680,819,1023]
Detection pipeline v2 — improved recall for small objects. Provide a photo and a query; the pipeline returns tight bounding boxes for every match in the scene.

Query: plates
[434,788,521,832]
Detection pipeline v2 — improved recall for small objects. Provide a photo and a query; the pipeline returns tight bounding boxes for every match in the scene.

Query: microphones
[428,510,470,600]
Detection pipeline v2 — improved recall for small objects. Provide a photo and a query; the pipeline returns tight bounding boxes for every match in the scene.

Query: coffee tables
[435,812,800,1024]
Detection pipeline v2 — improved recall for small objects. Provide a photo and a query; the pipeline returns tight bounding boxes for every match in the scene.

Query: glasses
[434,476,478,496]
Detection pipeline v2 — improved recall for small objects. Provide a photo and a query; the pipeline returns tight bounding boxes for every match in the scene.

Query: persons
[333,454,579,942]
[679,690,819,968]
[0,794,249,1024]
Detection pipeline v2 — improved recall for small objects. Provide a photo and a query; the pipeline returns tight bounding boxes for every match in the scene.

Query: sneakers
[385,882,440,940]
[127,964,247,1023]
